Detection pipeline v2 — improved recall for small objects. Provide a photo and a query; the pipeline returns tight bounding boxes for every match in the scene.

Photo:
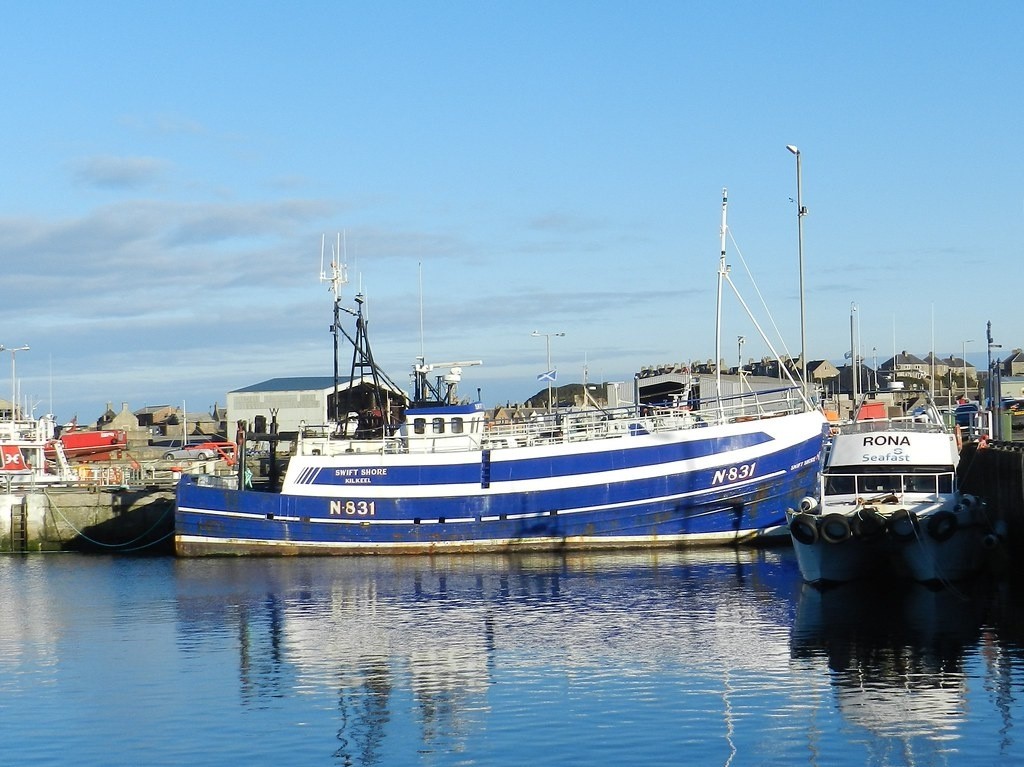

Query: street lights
[849,301,857,399]
[1,346,29,442]
[962,339,975,398]
[787,144,807,410]
[737,336,748,415]
[530,331,565,414]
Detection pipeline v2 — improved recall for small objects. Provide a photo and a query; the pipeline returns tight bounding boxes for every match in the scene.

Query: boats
[171,234,832,557]
[784,390,983,589]
[42,423,126,463]
[0,380,80,487]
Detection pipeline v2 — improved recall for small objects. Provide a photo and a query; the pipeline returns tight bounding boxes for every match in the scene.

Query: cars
[162,444,218,461]
[991,398,1024,427]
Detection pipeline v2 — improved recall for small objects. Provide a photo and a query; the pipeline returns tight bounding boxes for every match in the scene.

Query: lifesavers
[790,509,958,546]
[111,438,118,444]
